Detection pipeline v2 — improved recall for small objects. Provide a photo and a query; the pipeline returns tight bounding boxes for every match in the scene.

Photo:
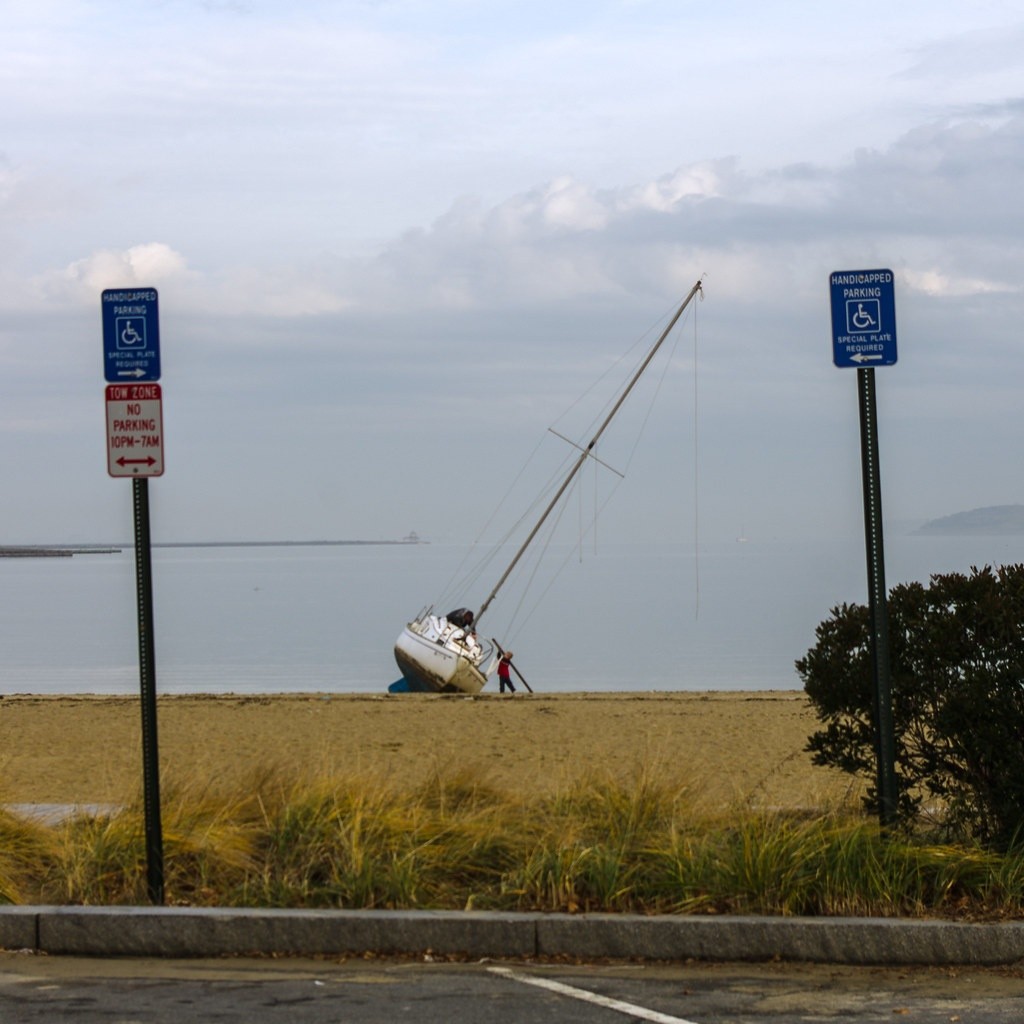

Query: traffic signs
[829,268,899,369]
[101,287,162,384]
[105,381,165,478]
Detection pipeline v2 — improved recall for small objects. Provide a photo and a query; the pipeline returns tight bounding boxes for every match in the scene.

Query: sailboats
[387,271,705,695]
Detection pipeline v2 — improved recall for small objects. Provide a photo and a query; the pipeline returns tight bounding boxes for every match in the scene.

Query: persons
[497,651,516,693]
[446,607,478,642]
